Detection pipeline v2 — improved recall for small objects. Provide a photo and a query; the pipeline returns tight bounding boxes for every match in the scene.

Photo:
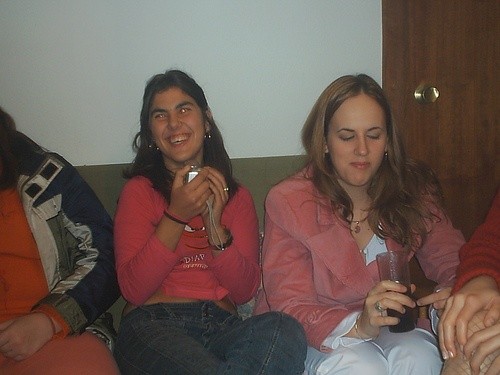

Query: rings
[224,187,230,191]
[375,302,384,311]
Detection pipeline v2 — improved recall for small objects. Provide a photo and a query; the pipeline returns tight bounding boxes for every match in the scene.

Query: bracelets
[355,315,363,340]
[164,208,189,224]
[44,312,56,335]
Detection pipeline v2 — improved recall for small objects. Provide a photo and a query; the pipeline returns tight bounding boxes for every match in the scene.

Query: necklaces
[349,216,368,232]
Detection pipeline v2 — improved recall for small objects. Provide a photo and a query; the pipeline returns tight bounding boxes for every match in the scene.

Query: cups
[377,247,417,333]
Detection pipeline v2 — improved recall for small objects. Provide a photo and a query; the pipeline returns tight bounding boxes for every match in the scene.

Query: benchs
[72,155,312,329]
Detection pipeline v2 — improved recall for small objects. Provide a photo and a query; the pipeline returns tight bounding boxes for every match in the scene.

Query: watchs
[211,229,233,250]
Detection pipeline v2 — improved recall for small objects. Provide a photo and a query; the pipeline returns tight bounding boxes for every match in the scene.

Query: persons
[0,110,118,375]
[438,185,500,374]
[113,71,307,375]
[252,75,469,375]
